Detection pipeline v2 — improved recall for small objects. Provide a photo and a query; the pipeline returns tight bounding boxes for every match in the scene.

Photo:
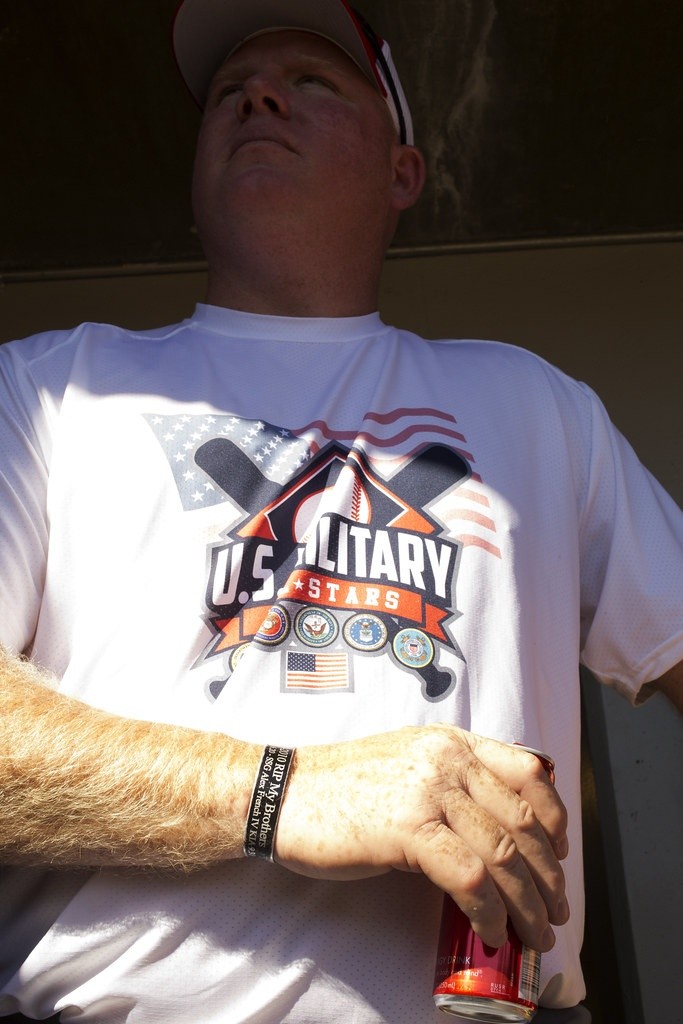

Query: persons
[0,0,683,1024]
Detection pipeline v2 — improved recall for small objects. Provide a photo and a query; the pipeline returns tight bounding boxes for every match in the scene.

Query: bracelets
[242,744,297,865]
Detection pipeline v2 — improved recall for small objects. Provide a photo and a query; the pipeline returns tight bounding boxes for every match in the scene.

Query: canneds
[431,742,555,1024]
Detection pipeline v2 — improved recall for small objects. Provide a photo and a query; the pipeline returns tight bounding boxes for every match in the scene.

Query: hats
[174,1,415,149]
